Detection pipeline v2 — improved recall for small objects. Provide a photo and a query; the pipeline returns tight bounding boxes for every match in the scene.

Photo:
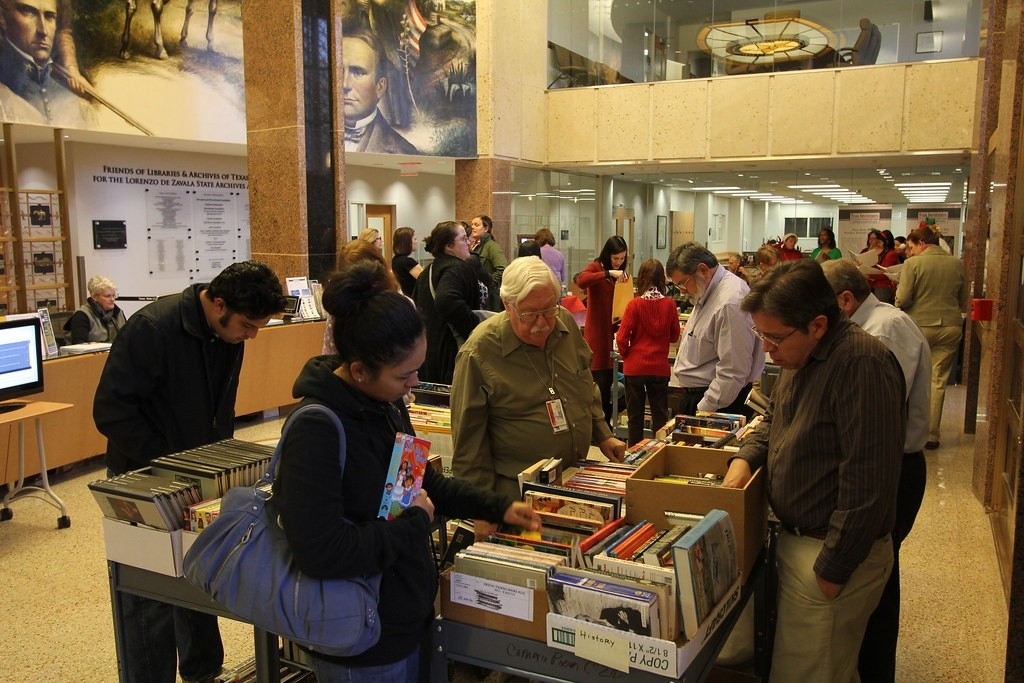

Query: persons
[616,259,681,449]
[665,241,766,425]
[819,259,932,683]
[894,227,967,450]
[725,223,952,306]
[62,274,126,345]
[92,258,289,683]
[576,234,628,434]
[321,213,567,386]
[721,257,908,683]
[443,255,626,543]
[272,260,542,683]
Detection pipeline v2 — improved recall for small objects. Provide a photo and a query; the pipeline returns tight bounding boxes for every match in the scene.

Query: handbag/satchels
[182,402,384,658]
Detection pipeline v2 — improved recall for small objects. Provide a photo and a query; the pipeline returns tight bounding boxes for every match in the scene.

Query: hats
[783,232,798,244]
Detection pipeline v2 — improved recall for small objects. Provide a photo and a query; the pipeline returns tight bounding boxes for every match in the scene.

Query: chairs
[826,18,881,66]
[547,40,635,89]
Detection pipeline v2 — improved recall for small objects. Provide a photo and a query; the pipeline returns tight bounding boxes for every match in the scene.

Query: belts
[779,525,830,540]
[669,386,709,395]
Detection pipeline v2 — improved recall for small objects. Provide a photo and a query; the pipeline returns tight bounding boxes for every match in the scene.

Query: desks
[609,353,675,438]
[0,319,338,486]
[0,399,74,529]
[107,559,759,683]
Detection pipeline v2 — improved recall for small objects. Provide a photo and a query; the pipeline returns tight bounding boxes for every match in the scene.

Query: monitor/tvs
[0,318,44,414]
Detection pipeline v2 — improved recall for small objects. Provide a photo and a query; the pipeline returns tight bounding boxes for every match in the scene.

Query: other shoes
[926,441,939,450]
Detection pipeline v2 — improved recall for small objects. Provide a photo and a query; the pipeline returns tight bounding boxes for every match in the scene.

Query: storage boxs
[545,571,744,679]
[182,529,199,557]
[439,566,549,641]
[102,515,184,578]
[626,445,762,587]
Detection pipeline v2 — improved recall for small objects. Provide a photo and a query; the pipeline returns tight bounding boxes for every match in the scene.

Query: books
[442,411,765,642]
[402,402,452,429]
[86,438,277,531]
[744,388,773,418]
[376,431,432,523]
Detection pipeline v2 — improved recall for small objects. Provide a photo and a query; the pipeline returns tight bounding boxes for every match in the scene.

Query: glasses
[512,302,560,320]
[376,236,381,241]
[750,311,828,347]
[452,236,468,243]
[674,272,692,290]
[97,292,120,298]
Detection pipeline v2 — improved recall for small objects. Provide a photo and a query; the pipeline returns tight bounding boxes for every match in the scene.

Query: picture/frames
[915,30,944,54]
[656,215,667,249]
